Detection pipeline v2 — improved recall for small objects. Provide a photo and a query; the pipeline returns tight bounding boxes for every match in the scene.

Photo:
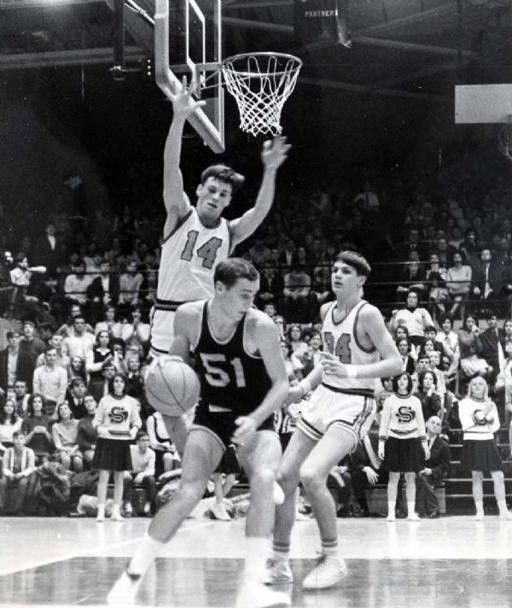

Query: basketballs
[145,360,201,417]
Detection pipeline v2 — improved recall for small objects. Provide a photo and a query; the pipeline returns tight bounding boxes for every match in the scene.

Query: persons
[262,248,405,593]
[0,158,510,518]
[143,70,293,510]
[97,253,297,608]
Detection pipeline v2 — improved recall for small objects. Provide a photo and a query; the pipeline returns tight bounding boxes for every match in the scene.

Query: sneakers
[261,556,293,584]
[499,511,511,520]
[232,581,292,608]
[110,512,128,522]
[406,512,421,521]
[108,569,145,605]
[473,510,486,521]
[210,503,234,523]
[304,551,350,590]
[94,512,108,525]
[385,513,399,523]
